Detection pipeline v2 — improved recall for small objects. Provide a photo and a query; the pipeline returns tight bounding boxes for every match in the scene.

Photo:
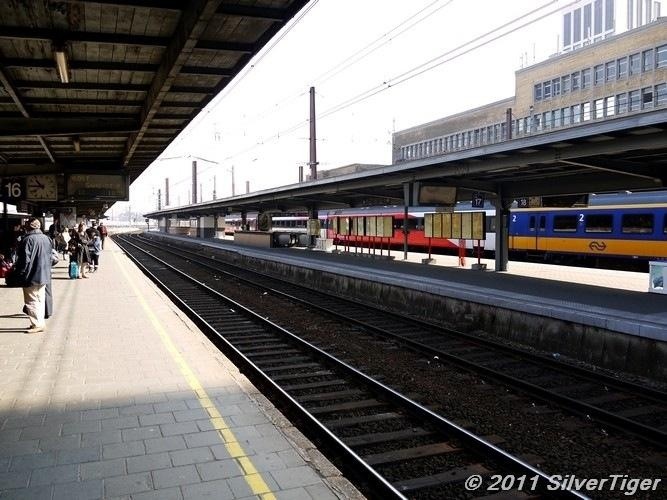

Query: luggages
[68,255,79,279]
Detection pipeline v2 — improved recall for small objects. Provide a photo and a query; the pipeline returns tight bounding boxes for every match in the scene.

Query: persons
[17,218,51,333]
[23,218,54,319]
[46,217,108,281]
[0,254,11,271]
[8,223,22,267]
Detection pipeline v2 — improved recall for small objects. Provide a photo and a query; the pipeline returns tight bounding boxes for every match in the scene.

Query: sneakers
[24,326,45,334]
[79,266,99,279]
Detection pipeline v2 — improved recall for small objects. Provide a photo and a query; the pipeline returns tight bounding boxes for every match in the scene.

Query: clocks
[23,173,59,203]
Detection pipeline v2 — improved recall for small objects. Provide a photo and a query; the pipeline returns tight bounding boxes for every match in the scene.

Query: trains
[222,187,667,274]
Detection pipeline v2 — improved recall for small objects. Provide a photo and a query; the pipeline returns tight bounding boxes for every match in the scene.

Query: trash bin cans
[215,227,225,237]
